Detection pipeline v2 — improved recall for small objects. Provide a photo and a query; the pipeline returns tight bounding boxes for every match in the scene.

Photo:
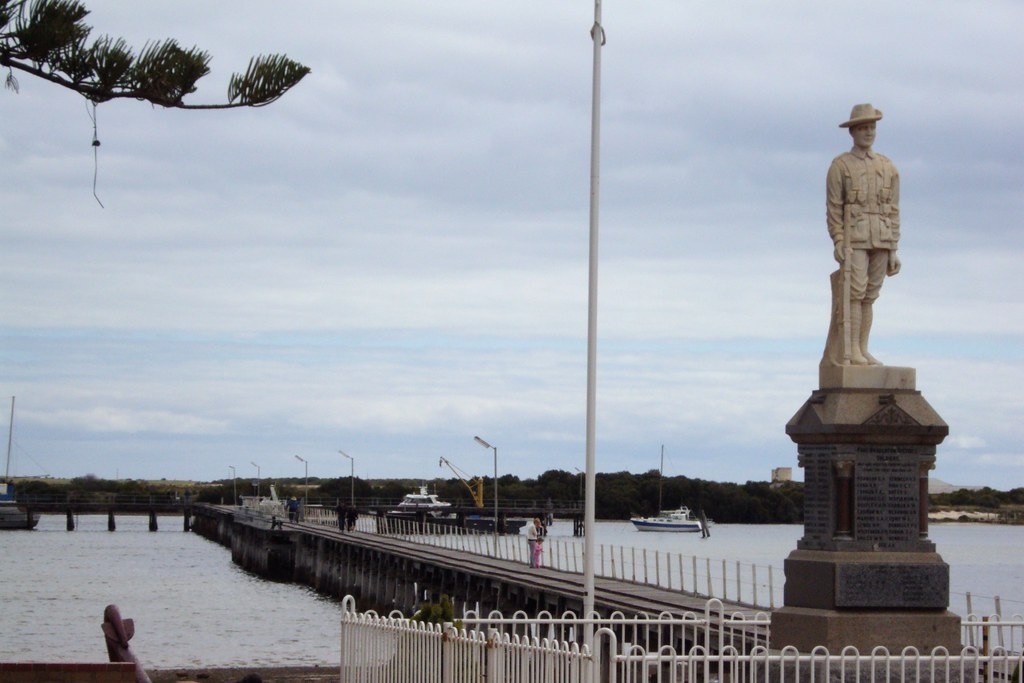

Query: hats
[291,496,297,500]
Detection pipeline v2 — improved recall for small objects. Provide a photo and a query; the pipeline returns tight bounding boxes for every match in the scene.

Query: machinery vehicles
[439,456,484,508]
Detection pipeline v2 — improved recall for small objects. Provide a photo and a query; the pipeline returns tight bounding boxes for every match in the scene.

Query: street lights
[251,462,260,496]
[474,436,498,558]
[295,455,307,504]
[339,450,354,508]
[229,466,236,507]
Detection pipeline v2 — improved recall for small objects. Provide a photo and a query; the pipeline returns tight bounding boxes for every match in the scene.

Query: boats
[630,444,715,531]
[0,397,40,529]
[397,486,451,507]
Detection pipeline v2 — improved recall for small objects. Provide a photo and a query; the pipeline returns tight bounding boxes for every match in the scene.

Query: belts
[528,539,536,541]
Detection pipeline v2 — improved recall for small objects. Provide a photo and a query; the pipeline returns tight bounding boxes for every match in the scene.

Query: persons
[527,518,544,568]
[284,497,300,524]
[826,103,901,366]
[335,500,358,534]
